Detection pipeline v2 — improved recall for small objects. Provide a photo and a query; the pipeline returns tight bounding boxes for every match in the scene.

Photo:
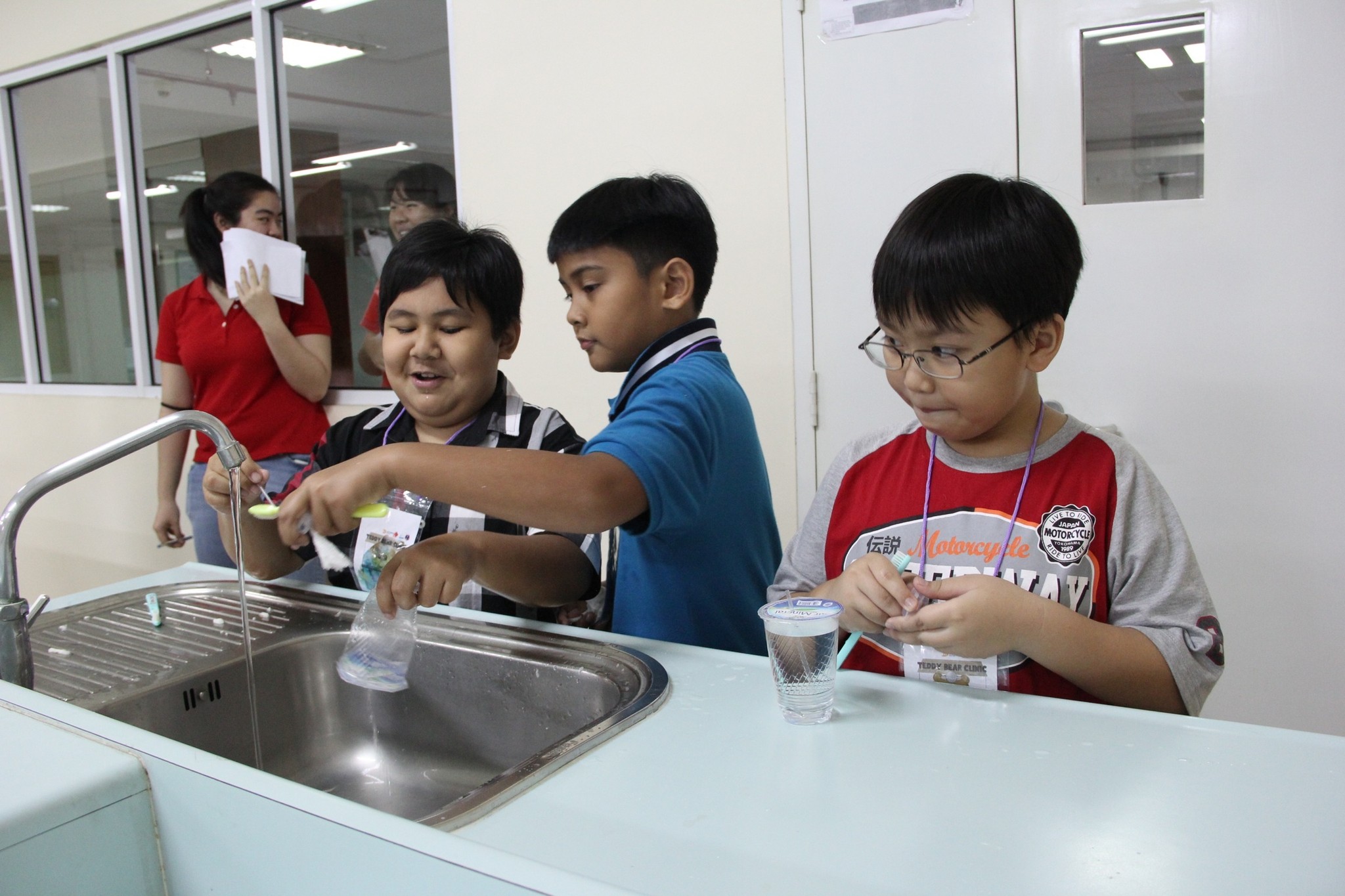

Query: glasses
[856,316,1039,379]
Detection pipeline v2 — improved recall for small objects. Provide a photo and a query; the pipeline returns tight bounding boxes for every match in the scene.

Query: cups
[757,597,842,725]
[336,586,417,693]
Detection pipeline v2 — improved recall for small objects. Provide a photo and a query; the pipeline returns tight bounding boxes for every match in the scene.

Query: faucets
[0,409,248,694]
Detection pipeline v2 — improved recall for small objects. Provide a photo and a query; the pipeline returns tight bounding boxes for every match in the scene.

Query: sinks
[99,624,669,833]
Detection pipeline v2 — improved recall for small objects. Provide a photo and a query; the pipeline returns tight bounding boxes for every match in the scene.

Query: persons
[357,160,459,391]
[153,172,332,586]
[766,171,1227,719]
[276,170,783,658]
[201,218,601,626]
